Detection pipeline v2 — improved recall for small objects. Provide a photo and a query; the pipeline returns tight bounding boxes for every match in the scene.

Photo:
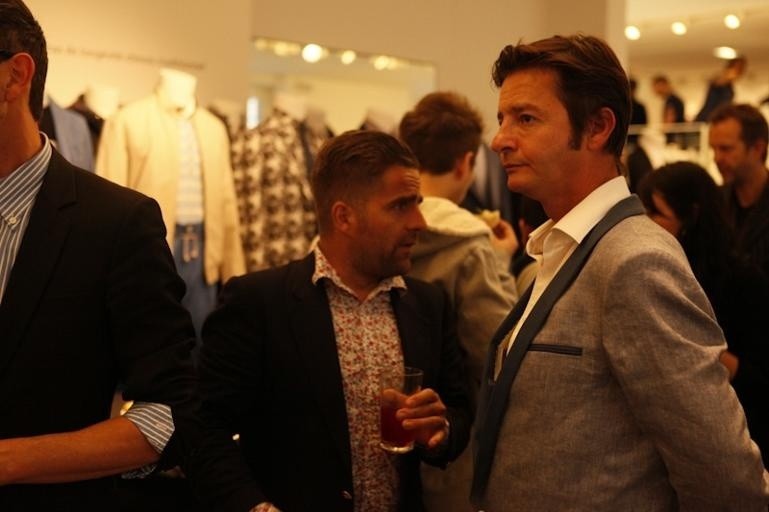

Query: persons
[0,0,205,510]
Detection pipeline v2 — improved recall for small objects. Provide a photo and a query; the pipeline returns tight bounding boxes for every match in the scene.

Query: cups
[379,365,424,455]
